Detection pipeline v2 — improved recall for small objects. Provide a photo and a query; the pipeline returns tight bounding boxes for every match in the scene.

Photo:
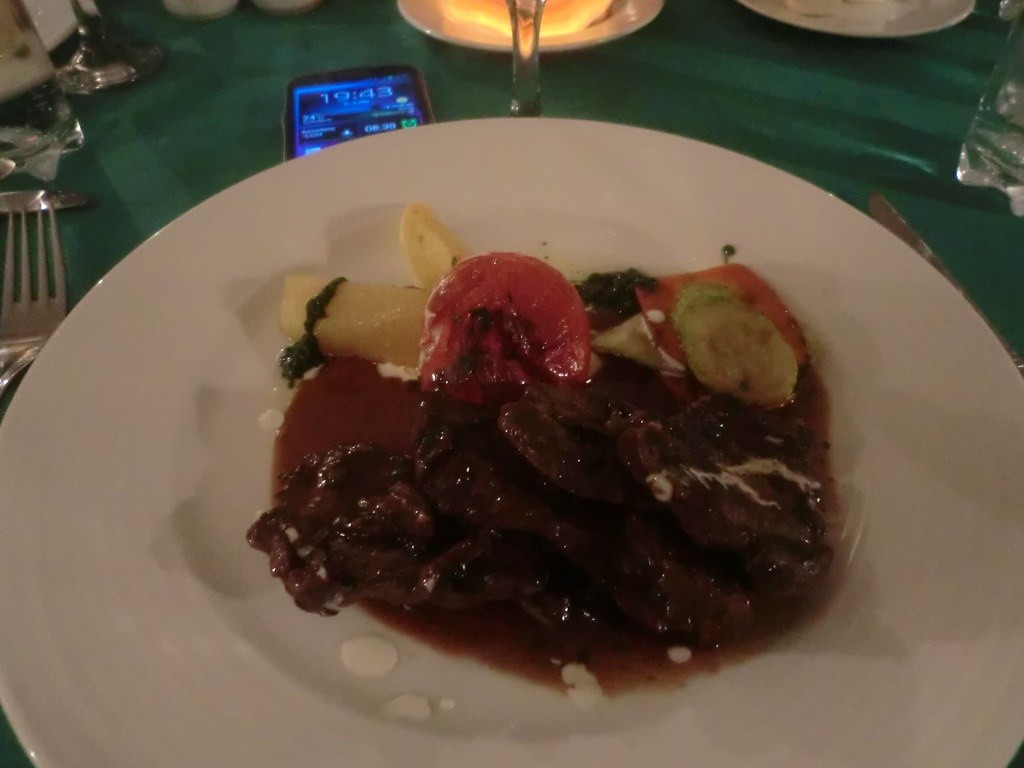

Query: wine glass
[54,0,170,95]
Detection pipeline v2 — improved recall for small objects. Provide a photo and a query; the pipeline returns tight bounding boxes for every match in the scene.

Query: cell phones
[281,66,436,163]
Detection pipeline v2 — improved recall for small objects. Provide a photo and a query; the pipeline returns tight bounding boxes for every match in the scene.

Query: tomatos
[636,264,809,385]
[419,251,592,409]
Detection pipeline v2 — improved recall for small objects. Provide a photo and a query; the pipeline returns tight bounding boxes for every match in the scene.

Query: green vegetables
[279,266,660,385]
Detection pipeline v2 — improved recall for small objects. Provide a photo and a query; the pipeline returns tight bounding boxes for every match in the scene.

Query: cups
[163,0,324,22]
[956,1,1024,217]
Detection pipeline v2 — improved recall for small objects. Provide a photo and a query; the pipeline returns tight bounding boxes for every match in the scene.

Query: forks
[0,201,68,396]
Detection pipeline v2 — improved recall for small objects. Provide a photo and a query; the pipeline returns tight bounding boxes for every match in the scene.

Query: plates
[397,0,665,54]
[734,0,978,40]
[0,113,1023,768]
